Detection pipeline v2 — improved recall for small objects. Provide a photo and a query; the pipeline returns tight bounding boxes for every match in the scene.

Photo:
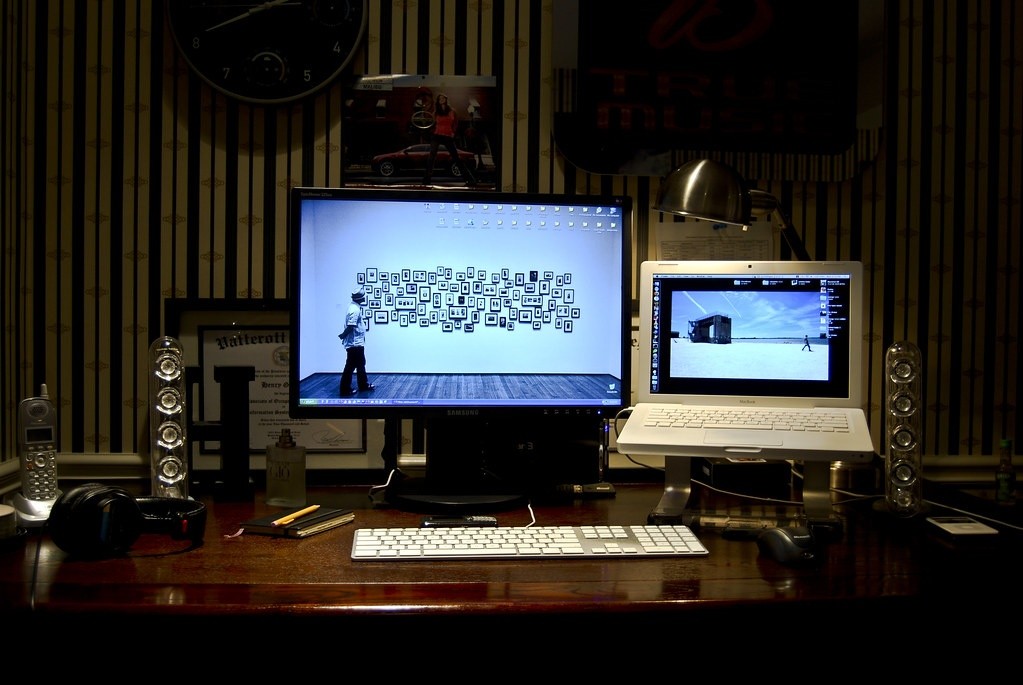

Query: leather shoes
[360,384,375,392]
[340,390,357,396]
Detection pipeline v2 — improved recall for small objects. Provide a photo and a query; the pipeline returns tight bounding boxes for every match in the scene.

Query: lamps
[653,157,811,261]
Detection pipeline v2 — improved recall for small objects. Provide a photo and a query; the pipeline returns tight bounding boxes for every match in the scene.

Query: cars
[373,145,478,178]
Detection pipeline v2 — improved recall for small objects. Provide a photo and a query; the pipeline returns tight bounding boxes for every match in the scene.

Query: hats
[352,287,364,299]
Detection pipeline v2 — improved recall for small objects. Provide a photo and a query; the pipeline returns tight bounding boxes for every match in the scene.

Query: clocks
[168,0,368,105]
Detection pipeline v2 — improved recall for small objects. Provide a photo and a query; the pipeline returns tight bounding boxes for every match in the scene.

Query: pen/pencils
[271,504,321,528]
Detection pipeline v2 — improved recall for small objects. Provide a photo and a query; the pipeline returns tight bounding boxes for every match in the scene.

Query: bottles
[265,429,307,508]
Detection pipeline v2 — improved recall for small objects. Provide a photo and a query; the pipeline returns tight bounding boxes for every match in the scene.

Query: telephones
[1,381,65,530]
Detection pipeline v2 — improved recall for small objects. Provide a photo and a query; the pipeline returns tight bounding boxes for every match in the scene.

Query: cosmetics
[266,428,306,507]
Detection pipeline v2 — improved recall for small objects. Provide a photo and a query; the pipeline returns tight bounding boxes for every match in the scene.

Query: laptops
[616,258,875,464]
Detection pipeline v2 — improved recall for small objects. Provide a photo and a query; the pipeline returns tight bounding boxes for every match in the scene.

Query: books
[239,507,356,538]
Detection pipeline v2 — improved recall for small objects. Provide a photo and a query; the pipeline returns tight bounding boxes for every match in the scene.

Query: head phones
[46,481,209,557]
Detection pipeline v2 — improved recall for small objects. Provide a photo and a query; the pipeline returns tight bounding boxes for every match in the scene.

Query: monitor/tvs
[287,188,633,520]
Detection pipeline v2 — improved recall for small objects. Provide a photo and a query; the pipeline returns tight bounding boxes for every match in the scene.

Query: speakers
[882,341,926,521]
[147,335,189,500]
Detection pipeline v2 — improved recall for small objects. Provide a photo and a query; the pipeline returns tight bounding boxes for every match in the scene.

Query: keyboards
[350,525,711,562]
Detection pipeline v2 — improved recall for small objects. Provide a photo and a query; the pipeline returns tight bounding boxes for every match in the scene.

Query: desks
[0,484,956,621]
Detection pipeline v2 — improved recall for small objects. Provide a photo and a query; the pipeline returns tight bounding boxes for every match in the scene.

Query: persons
[338,288,375,396]
[802,335,811,351]
[424,93,474,184]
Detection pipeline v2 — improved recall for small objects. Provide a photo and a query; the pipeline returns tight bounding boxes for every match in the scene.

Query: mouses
[758,525,821,569]
